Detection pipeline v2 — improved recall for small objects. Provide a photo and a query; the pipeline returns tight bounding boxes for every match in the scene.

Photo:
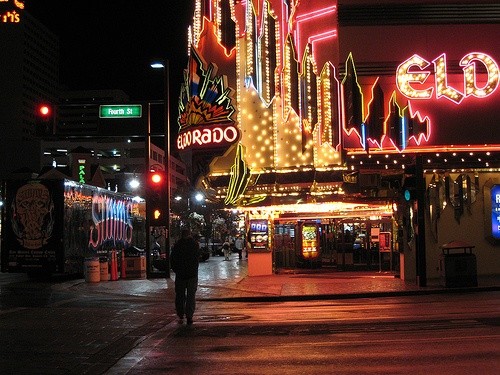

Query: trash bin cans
[438,240,478,287]
[83,256,100,283]
[100,255,109,282]
[119,246,146,278]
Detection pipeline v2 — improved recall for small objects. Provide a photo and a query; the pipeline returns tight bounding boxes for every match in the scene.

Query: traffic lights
[147,172,168,226]
[40,106,49,115]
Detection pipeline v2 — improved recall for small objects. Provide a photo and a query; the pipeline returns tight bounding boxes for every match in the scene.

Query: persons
[170,230,200,325]
[222,239,230,260]
[235,237,244,259]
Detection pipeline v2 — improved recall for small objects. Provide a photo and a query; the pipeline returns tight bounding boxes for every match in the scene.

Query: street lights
[148,56,171,280]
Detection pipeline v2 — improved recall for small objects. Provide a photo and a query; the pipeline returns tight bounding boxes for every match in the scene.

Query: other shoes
[187,319,193,325]
[179,317,184,319]
